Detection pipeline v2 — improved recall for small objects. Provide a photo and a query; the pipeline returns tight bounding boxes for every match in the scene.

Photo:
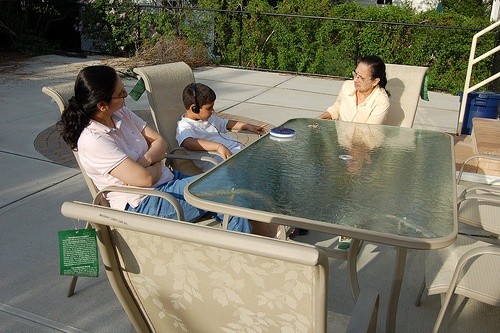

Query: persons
[316,56,390,125]
[62,65,297,238]
[176,83,308,236]
[333,119,386,173]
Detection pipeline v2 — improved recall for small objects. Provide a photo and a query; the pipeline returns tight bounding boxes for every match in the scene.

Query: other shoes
[295,228,309,235]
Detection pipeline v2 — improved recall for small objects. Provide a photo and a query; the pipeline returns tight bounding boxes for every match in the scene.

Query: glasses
[352,71,376,82]
[110,88,128,100]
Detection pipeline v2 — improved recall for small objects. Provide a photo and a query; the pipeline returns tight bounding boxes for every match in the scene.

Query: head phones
[191,84,200,113]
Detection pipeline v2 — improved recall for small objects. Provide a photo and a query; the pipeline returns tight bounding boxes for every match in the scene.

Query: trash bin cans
[459,90,500,135]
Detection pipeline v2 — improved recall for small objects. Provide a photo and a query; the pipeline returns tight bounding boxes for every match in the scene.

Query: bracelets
[217,144,223,150]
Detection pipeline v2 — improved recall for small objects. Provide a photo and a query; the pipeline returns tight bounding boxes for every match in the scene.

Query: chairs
[416,194,500,333]
[457,151,500,236]
[131,62,272,168]
[382,63,428,127]
[39,77,220,297]
[60,197,382,333]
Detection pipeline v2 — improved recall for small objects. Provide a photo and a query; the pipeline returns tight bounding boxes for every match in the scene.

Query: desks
[182,118,461,333]
